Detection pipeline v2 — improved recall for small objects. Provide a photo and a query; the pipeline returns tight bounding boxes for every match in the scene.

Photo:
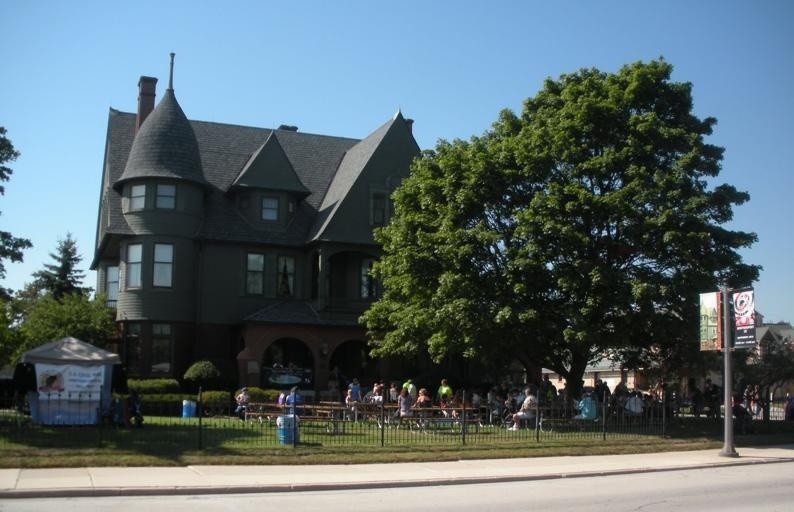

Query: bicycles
[127,386,144,427]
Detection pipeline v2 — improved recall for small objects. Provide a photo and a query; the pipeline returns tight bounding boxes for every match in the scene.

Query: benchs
[241,383,725,435]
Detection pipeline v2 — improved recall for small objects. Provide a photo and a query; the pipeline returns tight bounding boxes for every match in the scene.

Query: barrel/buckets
[275,414,300,444]
[182,400,196,417]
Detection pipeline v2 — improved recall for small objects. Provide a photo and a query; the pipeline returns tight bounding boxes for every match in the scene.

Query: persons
[562,377,765,431]
[286,385,304,416]
[234,386,251,416]
[126,386,141,425]
[346,377,559,431]
